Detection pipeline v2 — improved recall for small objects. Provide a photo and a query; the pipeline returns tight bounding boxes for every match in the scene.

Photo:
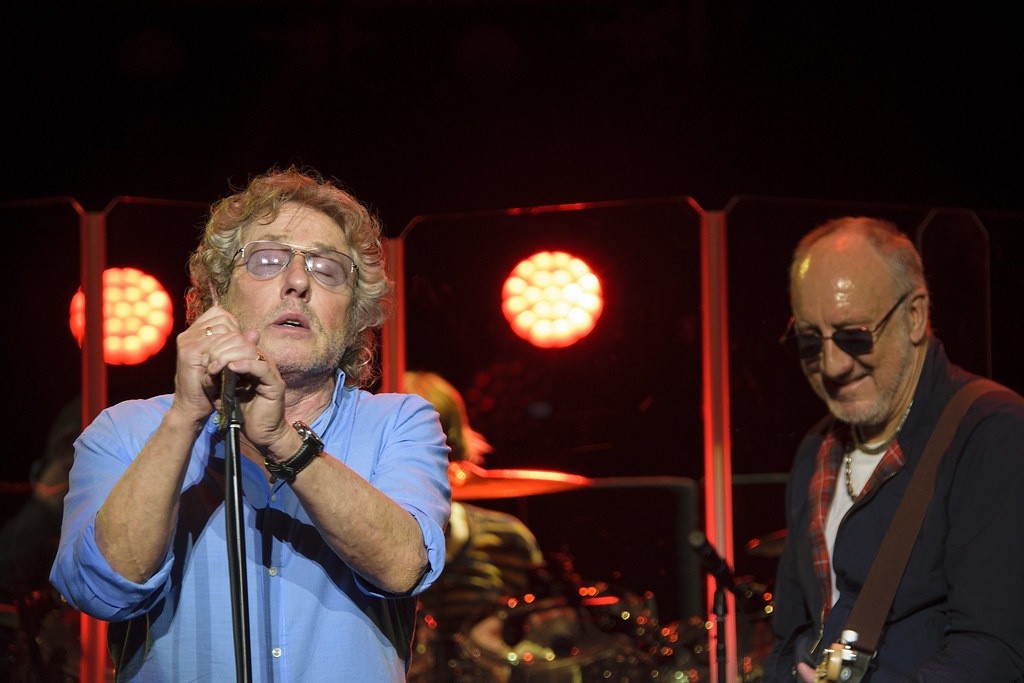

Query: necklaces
[839,455,867,506]
[847,397,913,455]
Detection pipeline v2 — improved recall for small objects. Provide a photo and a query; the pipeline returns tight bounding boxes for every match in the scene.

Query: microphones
[688,531,746,600]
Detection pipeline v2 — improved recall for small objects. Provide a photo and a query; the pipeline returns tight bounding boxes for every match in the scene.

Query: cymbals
[449,460,590,501]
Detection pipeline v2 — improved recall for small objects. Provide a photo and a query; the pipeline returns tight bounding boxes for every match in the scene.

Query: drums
[466,590,646,683]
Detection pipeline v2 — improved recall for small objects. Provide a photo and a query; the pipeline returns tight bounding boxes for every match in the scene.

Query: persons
[48,167,453,683]
[767,216,1024,683]
[399,371,545,682]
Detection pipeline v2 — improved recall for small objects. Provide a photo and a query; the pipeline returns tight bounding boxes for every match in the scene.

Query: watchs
[263,421,325,481]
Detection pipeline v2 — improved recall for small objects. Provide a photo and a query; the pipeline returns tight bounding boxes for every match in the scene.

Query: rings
[206,326,213,336]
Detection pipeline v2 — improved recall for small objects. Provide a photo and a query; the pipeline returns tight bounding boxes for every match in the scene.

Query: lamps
[497,244,622,363]
[62,256,189,376]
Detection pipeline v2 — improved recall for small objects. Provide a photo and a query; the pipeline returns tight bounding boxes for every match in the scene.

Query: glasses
[779,291,912,358]
[228,241,359,289]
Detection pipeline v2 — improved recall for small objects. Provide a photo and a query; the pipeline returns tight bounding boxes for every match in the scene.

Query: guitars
[814,629,859,683]
